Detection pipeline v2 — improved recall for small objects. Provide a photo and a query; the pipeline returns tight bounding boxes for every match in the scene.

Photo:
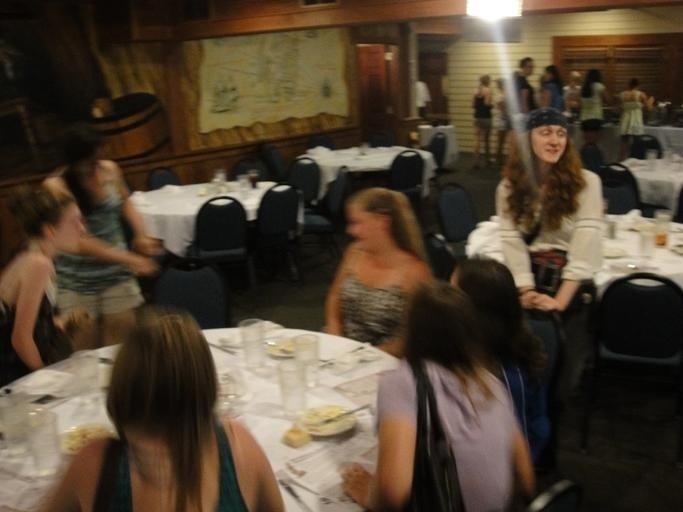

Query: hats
[527,107,566,128]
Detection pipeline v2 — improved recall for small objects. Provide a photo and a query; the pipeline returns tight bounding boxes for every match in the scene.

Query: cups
[638,220,656,260]
[240,319,265,368]
[654,209,673,247]
[22,412,60,474]
[291,334,319,389]
[278,360,307,419]
[661,149,673,170]
[248,169,258,188]
[71,350,101,419]
[2,408,33,474]
[646,150,658,173]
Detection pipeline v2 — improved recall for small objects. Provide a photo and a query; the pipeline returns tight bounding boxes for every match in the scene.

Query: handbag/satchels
[414,444,467,512]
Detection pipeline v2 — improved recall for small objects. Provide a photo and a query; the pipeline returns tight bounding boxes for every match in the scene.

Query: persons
[469,58,655,173]
[0,176,86,384]
[416,81,432,117]
[491,105,605,512]
[449,256,553,470]
[321,182,434,360]
[341,283,536,510]
[23,299,287,512]
[38,124,166,352]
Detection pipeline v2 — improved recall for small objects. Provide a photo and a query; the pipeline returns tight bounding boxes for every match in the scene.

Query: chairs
[154,261,230,330]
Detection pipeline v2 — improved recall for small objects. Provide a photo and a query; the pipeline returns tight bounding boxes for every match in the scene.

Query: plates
[298,406,356,437]
[60,422,115,458]
[265,338,295,358]
